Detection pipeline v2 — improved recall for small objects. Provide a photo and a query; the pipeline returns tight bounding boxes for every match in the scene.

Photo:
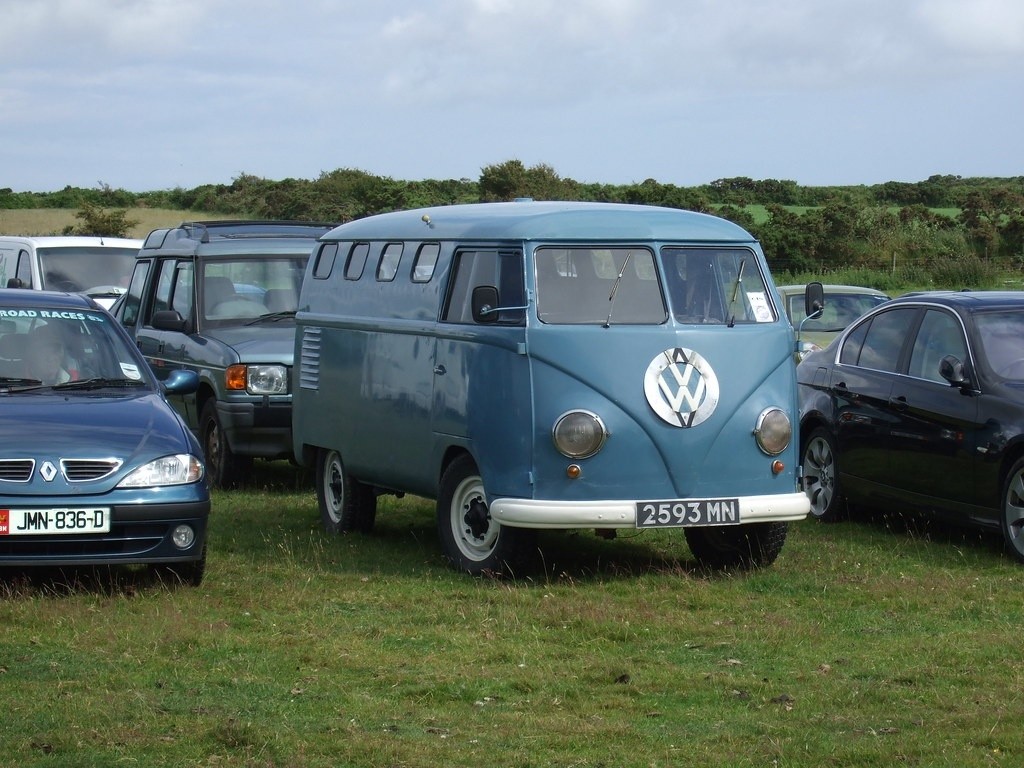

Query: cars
[794,290,1023,563]
[2,287,212,586]
[743,284,893,368]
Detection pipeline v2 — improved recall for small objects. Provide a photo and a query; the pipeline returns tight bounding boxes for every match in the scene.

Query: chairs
[196,275,303,321]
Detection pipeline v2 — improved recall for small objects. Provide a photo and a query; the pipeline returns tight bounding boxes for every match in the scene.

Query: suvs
[128,216,351,481]
[1,232,148,314]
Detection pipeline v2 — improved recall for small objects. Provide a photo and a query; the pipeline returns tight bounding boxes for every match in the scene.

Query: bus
[292,197,827,580]
